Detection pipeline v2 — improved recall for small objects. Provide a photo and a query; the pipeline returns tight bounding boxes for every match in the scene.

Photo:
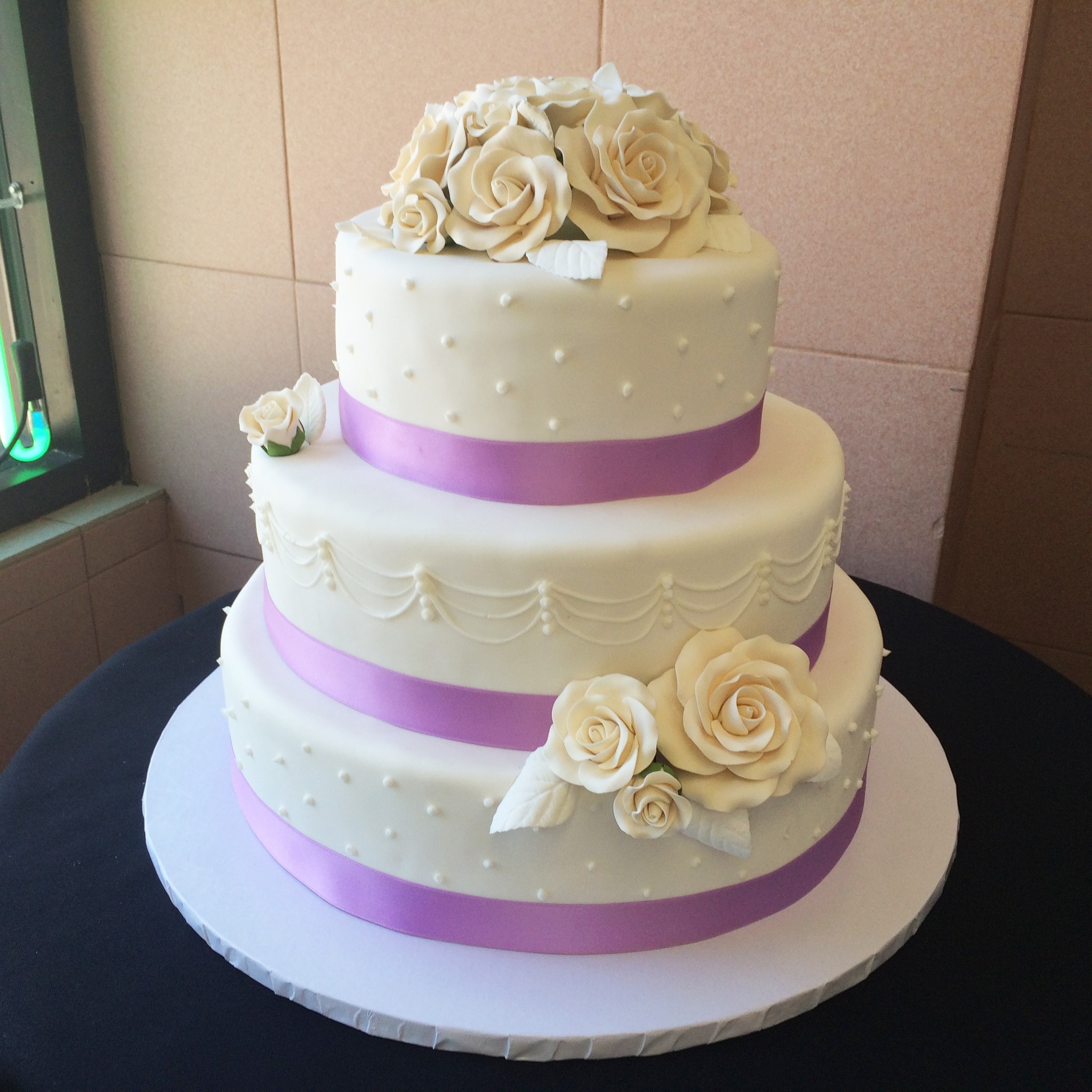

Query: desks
[0,572,1092,1092]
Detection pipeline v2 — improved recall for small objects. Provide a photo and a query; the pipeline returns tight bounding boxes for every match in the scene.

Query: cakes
[218,59,888,958]
